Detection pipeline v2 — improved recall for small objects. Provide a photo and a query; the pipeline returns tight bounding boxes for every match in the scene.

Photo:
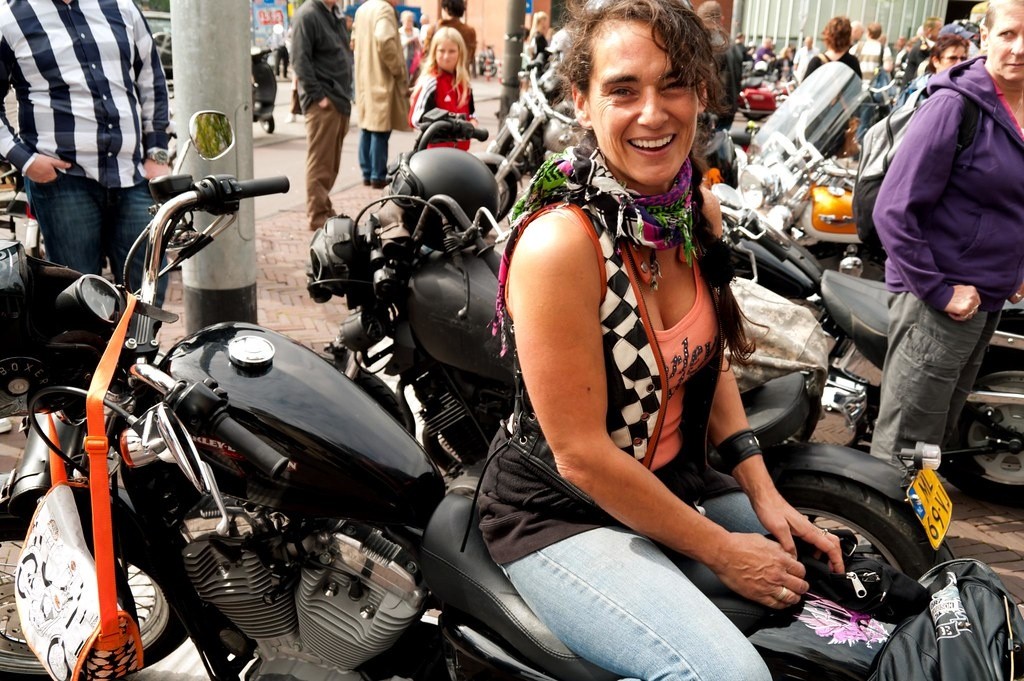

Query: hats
[938,24,975,41]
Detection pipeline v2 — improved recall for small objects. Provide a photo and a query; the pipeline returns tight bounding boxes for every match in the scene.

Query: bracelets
[714,426,763,477]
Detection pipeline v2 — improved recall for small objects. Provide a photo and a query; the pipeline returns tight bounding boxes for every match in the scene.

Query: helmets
[405,149,500,253]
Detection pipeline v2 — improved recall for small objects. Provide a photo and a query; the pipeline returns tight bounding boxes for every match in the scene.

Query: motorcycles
[0,25,1024,681]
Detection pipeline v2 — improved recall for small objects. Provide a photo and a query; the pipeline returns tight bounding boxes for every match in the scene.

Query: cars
[144,12,172,79]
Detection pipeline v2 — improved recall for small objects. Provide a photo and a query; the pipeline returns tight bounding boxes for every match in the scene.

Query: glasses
[387,150,424,208]
[938,56,967,64]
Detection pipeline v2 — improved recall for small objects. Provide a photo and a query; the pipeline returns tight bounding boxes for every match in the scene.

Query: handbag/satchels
[15,482,144,681]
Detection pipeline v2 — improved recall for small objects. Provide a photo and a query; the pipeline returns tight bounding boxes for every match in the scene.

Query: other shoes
[370,177,392,188]
[364,178,371,186]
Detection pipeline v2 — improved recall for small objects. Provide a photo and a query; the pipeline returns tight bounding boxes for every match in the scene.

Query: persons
[869,0,1024,472]
[409,24,479,152]
[287,0,356,232]
[2,0,174,408]
[423,0,478,75]
[523,0,988,160]
[349,0,414,187]
[416,11,431,46]
[396,8,421,95]
[269,21,290,80]
[474,1,850,681]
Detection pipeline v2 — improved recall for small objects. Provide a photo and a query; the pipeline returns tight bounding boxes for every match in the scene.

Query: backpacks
[853,84,980,262]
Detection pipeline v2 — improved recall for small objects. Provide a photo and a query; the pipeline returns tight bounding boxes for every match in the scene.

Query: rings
[821,528,829,537]
[974,303,979,309]
[775,586,788,600]
[1014,291,1021,299]
[969,309,974,314]
[960,313,968,319]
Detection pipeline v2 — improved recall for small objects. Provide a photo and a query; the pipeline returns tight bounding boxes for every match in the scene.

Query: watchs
[144,149,171,164]
